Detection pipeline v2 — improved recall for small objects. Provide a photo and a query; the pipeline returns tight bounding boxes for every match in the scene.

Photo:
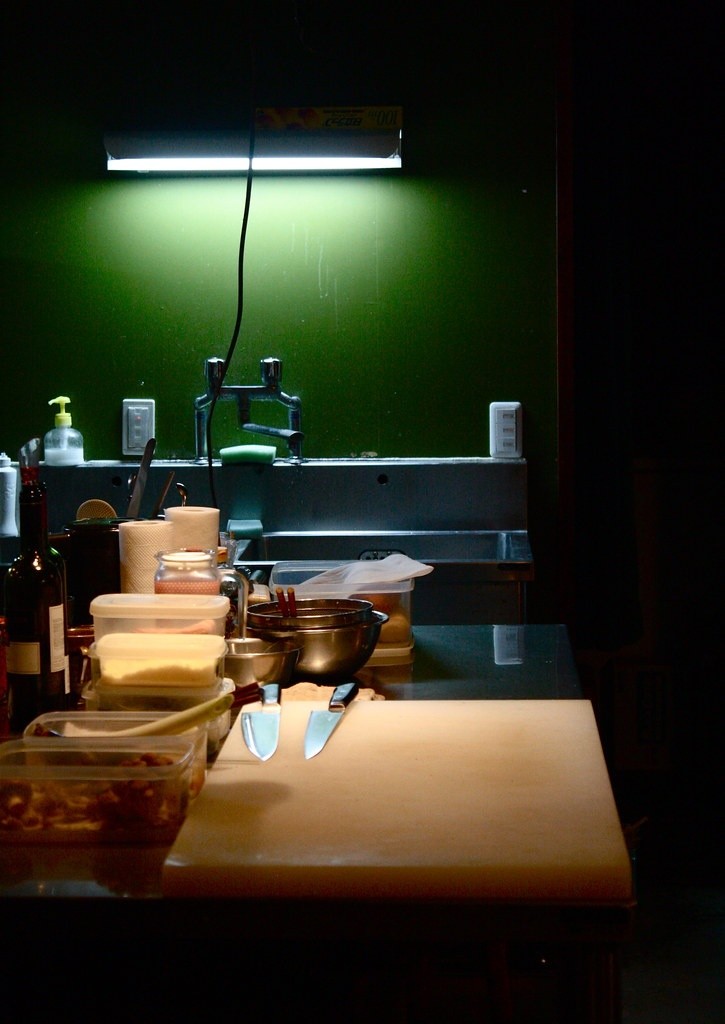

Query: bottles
[5,481,71,726]
[217,547,238,638]
[0,453,19,537]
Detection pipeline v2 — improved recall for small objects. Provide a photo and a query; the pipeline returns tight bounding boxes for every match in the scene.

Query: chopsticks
[276,588,297,616]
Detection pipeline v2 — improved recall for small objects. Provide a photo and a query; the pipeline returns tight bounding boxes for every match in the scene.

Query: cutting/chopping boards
[162,700,632,899]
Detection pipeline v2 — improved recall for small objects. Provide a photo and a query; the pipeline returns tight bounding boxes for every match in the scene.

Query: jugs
[154,548,219,628]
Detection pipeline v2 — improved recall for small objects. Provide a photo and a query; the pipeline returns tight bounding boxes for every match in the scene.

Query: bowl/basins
[207,716,222,756]
[247,599,389,678]
[224,638,306,686]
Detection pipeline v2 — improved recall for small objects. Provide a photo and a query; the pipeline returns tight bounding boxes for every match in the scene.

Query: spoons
[176,482,187,506]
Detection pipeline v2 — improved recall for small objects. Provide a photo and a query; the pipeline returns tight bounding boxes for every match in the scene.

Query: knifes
[126,438,156,518]
[304,683,359,759]
[241,683,280,761]
